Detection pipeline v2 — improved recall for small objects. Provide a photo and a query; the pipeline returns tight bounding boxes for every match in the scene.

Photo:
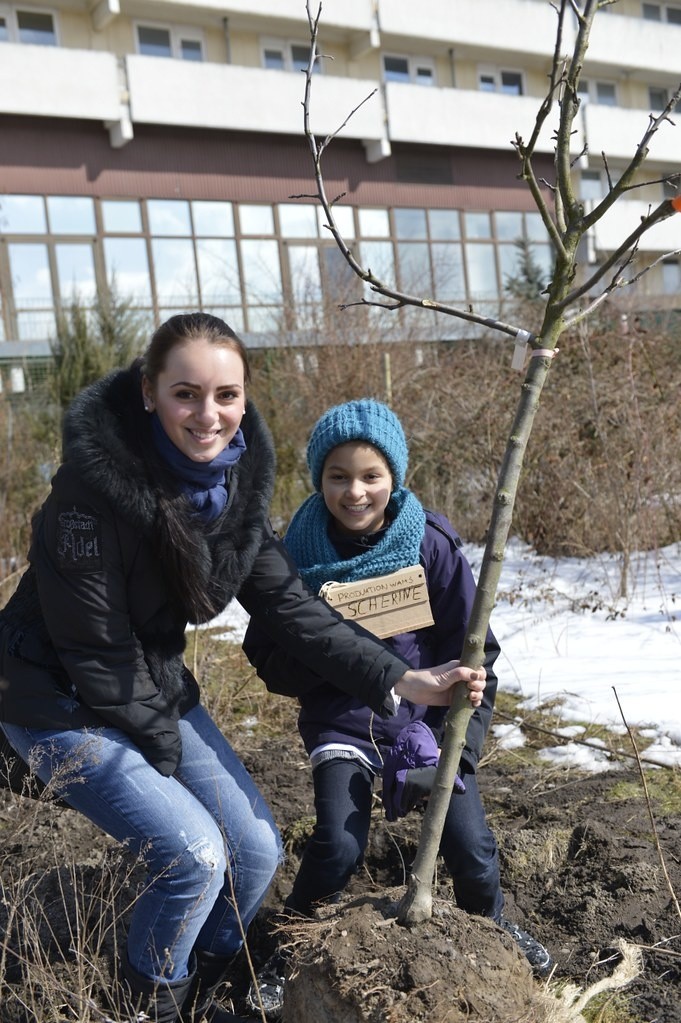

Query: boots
[120,938,197,1023]
[182,941,254,1023]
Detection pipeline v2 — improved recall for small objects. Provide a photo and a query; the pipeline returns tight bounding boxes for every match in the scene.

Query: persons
[0,313,488,1023]
[242,402,551,1020]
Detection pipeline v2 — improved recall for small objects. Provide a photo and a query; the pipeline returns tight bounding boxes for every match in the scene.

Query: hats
[306,400,409,497]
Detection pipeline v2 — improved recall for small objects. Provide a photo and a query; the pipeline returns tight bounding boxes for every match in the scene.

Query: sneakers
[476,911,550,968]
[248,948,300,1013]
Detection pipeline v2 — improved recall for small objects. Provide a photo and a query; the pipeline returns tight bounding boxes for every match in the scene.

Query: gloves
[382,720,468,824]
